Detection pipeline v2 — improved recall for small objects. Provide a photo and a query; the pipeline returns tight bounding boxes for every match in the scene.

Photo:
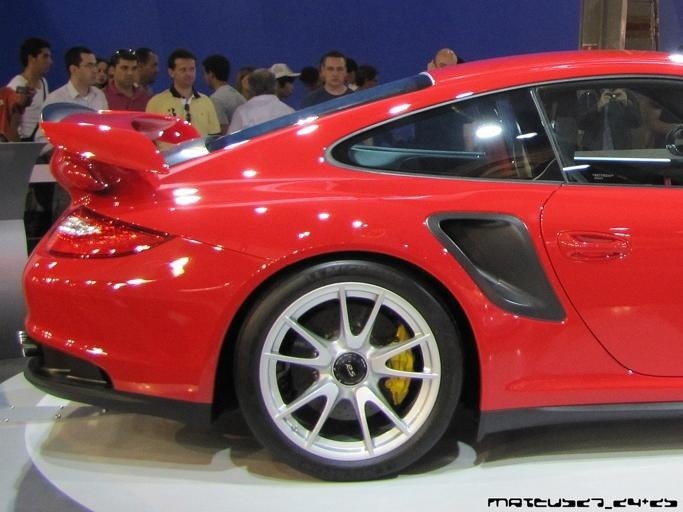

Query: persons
[0,35,682,239]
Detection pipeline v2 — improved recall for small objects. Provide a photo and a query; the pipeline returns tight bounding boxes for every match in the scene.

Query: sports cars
[15,48,682,476]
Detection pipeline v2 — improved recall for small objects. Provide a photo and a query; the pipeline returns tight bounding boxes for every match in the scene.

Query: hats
[268,63,301,79]
